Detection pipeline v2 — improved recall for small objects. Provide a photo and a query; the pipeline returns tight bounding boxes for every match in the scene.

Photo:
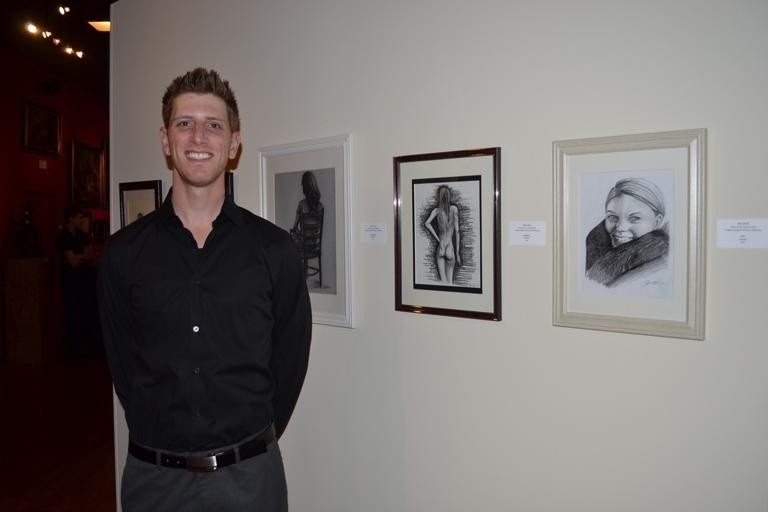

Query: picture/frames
[21,97,64,157]
[67,137,103,208]
[550,127,711,343]
[392,146,506,323]
[256,135,355,330]
[118,178,162,228]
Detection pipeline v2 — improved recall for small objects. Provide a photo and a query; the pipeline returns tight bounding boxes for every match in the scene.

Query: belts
[126,418,277,473]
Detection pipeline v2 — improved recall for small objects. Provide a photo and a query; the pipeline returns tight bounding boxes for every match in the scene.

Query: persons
[424,185,464,285]
[584,176,669,290]
[91,66,314,512]
[290,172,325,257]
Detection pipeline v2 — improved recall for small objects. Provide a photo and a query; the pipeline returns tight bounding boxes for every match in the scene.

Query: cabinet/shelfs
[4,257,66,368]
